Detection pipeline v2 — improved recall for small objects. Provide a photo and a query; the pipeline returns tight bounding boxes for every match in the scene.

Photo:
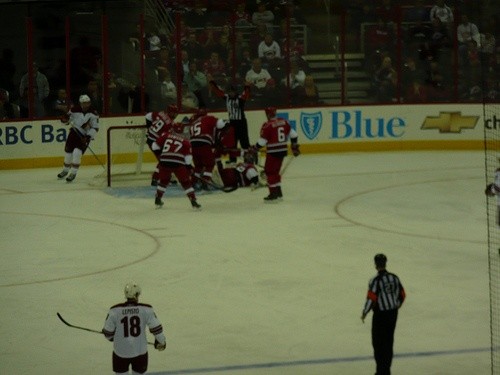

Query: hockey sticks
[57,312,155,346]
[265,153,293,187]
[191,172,240,194]
[68,122,106,169]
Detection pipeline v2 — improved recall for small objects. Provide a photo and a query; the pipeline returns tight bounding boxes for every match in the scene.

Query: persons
[102,282,166,375]
[361,254,407,375]
[0,0,312,210]
[340,0,500,105]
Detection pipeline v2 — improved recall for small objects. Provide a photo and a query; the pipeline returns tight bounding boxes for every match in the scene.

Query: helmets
[124,282,141,297]
[265,106,276,116]
[374,254,387,265]
[168,104,178,113]
[79,94,90,103]
[174,122,183,133]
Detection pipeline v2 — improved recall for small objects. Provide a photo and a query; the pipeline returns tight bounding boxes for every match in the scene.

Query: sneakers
[150,178,159,187]
[154,196,164,206]
[191,199,201,208]
[58,170,67,177]
[263,193,283,200]
[226,156,236,164]
[66,174,77,181]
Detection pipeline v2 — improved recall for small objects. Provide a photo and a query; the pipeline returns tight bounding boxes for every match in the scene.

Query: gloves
[248,145,257,154]
[84,135,91,144]
[62,113,71,122]
[291,145,299,156]
